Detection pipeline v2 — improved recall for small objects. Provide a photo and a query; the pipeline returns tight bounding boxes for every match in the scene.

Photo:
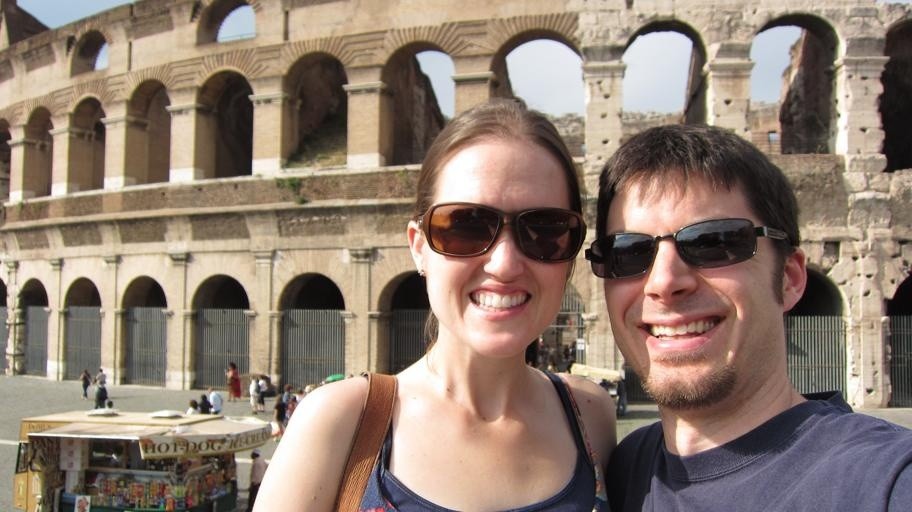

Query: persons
[78,360,307,512]
[527,340,576,372]
[253,104,617,511]
[584,125,909,511]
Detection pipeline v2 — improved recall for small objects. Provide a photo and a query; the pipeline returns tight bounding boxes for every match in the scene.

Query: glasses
[417,202,587,265]
[585,217,790,278]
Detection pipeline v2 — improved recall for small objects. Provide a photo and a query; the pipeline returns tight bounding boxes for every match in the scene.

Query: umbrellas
[324,371,345,380]
